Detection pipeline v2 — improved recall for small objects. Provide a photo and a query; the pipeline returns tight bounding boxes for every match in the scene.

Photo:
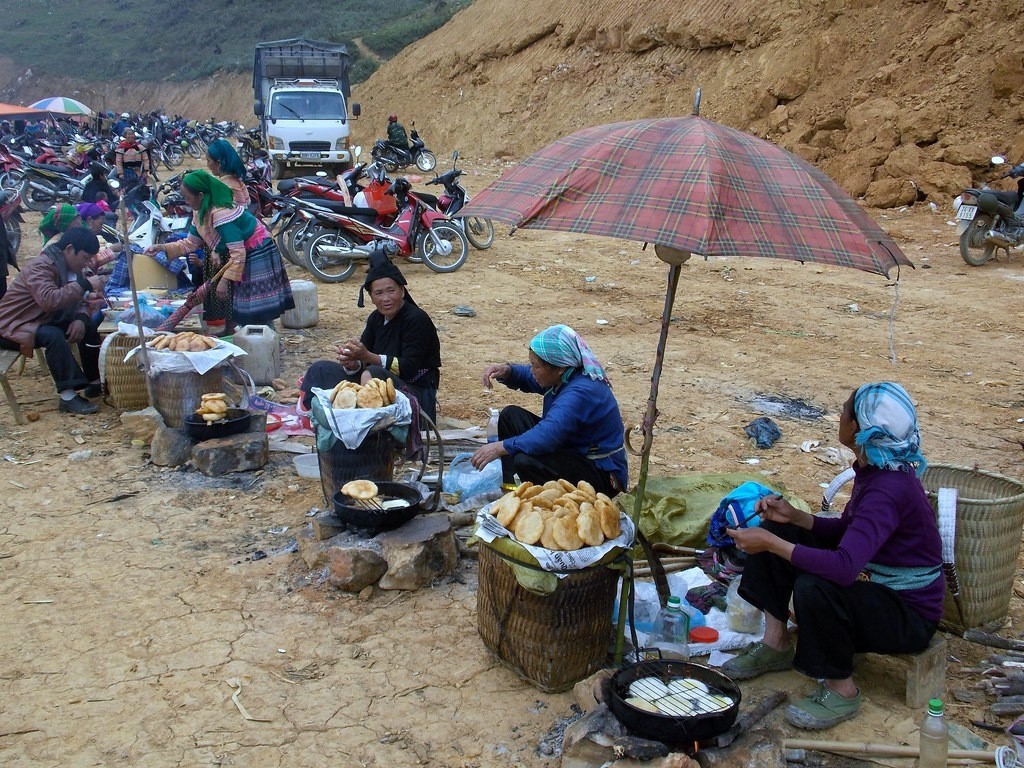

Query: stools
[852,639,947,709]
[0,348,49,425]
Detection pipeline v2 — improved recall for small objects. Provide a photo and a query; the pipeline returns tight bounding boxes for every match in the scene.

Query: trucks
[252,38,361,179]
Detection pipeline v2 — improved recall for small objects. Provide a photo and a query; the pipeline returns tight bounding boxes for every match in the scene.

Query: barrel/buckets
[233,325,280,385]
[280,279,319,328]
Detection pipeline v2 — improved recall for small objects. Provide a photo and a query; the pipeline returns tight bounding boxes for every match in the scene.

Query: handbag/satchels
[114,295,176,328]
[445,451,505,503]
[611,574,708,637]
[725,573,763,633]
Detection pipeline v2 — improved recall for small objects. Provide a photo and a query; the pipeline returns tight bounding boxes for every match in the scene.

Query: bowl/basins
[101,308,130,322]
[266,414,282,432]
[123,290,146,297]
[129,299,157,306]
[292,453,322,481]
[301,416,313,430]
[153,306,162,310]
[104,297,132,308]
[157,297,170,307]
[170,301,196,317]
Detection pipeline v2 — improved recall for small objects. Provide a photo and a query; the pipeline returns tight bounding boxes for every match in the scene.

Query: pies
[624,676,735,716]
[489,479,620,550]
[329,377,396,409]
[340,479,379,500]
[353,496,410,510]
[148,331,217,352]
[198,392,228,420]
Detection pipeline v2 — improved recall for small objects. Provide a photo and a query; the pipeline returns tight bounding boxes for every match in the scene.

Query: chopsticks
[87,272,113,308]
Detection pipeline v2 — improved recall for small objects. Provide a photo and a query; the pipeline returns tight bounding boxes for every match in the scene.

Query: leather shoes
[59,393,100,415]
[84,382,102,398]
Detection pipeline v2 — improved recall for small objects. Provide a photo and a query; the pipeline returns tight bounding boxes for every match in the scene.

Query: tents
[0,103,67,138]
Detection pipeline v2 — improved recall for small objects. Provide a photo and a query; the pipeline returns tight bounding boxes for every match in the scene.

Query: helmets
[121,112,130,121]
[388,115,397,123]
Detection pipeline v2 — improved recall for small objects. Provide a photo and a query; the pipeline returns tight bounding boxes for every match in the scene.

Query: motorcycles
[370,121,436,173]
[0,115,242,251]
[955,155,1024,266]
[236,124,262,164]
[268,144,494,283]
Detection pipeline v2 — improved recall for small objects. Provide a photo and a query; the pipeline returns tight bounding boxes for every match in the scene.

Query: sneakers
[784,685,862,731]
[722,641,795,681]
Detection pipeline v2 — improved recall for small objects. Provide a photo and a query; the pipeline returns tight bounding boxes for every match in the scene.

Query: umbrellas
[154,260,234,332]
[451,88,915,668]
[27,97,91,122]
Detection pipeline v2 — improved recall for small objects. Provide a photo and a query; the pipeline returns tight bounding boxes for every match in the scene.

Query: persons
[143,140,296,356]
[470,324,628,500]
[720,381,945,730]
[115,126,149,179]
[298,249,441,450]
[0,165,120,414]
[99,111,182,145]
[385,114,408,147]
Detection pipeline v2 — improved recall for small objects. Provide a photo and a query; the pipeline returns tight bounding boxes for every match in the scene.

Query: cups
[179,257,189,276]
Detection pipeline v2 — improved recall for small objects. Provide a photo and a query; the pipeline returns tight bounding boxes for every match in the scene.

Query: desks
[97,313,203,341]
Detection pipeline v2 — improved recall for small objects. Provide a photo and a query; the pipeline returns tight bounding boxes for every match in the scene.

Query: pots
[183,407,251,438]
[610,648,741,740]
[331,481,422,528]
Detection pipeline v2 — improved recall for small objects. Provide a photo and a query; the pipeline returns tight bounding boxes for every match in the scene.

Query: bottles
[644,596,690,661]
[919,699,947,768]
[487,407,499,443]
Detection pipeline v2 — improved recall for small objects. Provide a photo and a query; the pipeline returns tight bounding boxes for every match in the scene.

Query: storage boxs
[130,254,178,290]
